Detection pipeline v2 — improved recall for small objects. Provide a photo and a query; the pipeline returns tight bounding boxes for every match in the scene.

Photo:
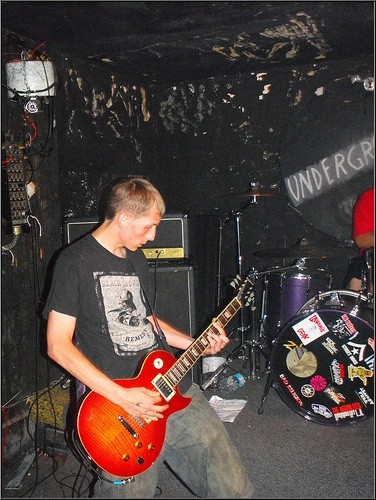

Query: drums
[272,291,375,427]
[265,269,333,346]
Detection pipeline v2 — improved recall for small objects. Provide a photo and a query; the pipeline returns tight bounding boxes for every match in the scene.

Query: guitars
[71,266,260,483]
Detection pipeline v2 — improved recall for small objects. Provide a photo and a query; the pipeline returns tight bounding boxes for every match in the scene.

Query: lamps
[5,60,55,114]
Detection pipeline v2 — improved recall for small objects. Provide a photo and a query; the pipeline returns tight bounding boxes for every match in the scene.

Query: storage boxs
[144,262,202,389]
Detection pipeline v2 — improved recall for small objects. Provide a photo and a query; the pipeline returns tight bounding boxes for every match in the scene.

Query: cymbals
[234,182,283,200]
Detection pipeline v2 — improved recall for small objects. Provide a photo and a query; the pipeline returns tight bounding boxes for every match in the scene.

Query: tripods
[201,199,300,389]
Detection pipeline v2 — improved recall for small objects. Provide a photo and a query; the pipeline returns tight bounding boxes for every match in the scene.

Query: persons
[345,187,375,291]
[43,177,255,499]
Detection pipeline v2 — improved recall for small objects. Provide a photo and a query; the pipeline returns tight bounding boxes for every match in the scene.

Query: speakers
[66,213,202,392]
